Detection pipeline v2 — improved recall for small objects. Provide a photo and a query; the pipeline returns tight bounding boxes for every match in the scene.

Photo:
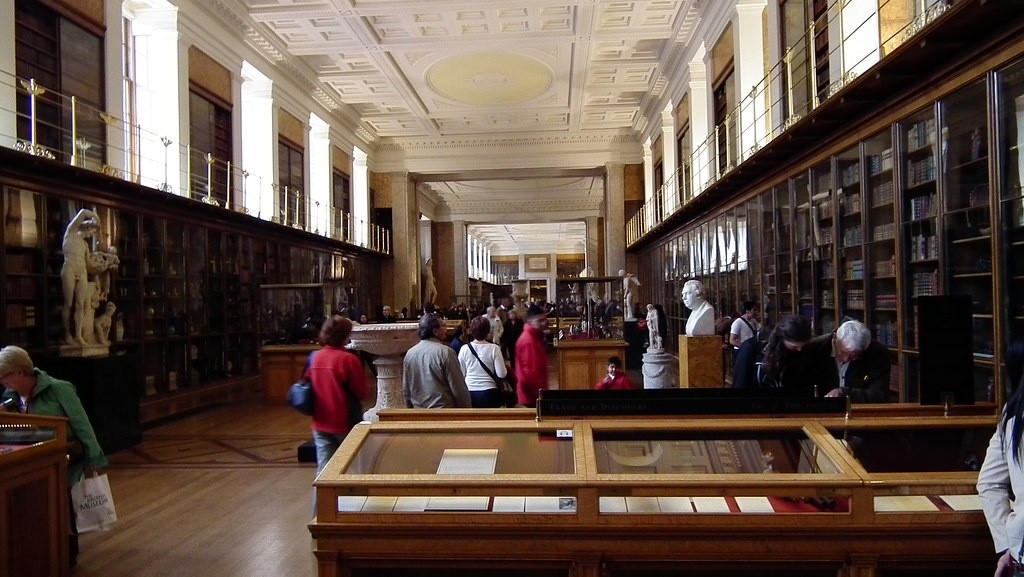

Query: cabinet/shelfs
[638,54,1024,415]
[263,354,307,404]
[562,355,622,390]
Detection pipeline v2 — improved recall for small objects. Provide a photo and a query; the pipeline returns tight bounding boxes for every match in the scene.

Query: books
[816,111,941,351]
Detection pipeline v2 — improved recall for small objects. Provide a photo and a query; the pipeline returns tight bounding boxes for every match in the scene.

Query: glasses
[835,341,858,359]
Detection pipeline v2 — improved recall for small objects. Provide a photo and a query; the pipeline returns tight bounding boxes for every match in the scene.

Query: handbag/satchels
[497,379,513,398]
[72,470,118,533]
[288,351,316,416]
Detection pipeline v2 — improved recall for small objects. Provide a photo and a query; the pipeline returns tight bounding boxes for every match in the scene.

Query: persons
[299,315,374,522]
[720,299,814,403]
[587,265,598,301]
[264,295,667,407]
[646,304,663,349]
[681,280,715,334]
[0,346,108,575]
[617,269,636,320]
[422,258,439,310]
[476,278,484,301]
[977,325,1024,577]
[60,208,120,348]
[799,320,891,402]
